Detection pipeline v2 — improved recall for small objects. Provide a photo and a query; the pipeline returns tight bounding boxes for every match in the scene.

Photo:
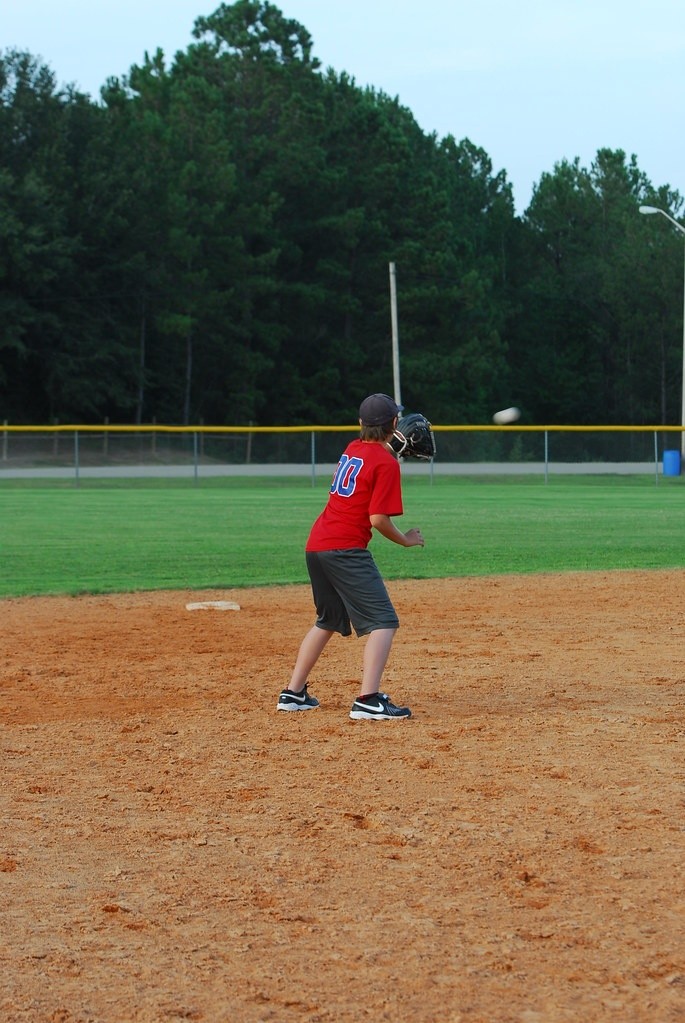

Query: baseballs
[497,408,519,422]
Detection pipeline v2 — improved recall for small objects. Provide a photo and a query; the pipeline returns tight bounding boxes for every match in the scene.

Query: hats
[360,393,405,427]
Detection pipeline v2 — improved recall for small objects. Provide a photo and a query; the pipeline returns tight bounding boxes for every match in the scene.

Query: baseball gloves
[384,412,436,462]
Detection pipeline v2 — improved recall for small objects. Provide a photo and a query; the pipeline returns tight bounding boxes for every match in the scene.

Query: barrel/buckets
[662,449,681,476]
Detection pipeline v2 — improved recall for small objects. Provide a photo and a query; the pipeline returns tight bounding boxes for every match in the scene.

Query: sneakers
[278,684,320,711]
[349,693,411,720]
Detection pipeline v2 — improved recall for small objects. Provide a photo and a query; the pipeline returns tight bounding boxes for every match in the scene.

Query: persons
[277,391,429,721]
